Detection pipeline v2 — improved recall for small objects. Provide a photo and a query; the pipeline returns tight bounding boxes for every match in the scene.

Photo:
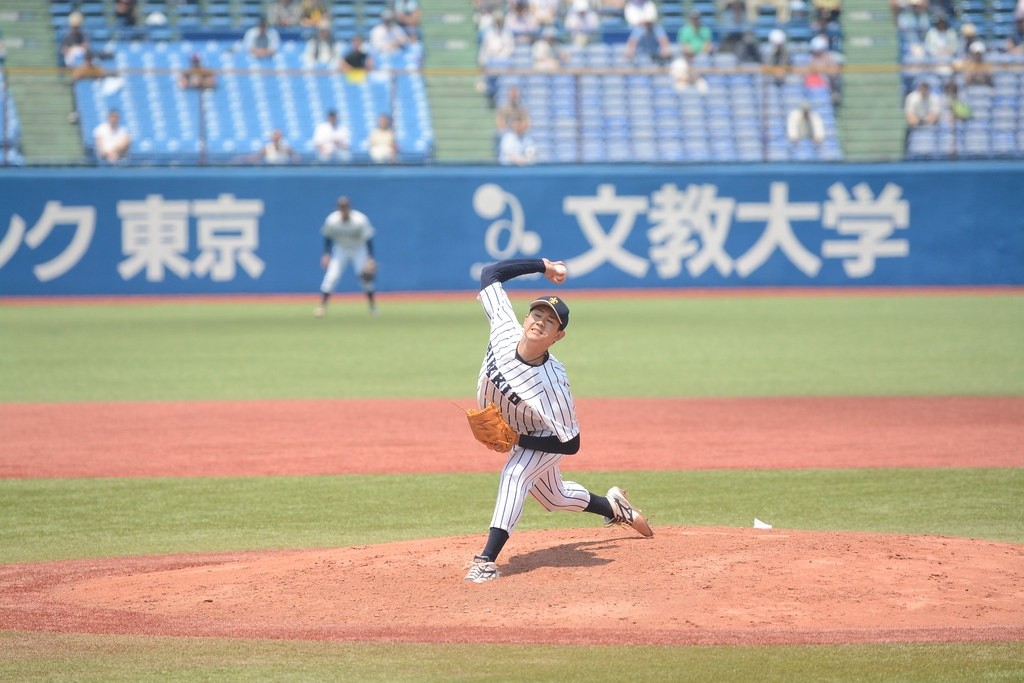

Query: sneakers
[603,486,653,538]
[461,555,498,584]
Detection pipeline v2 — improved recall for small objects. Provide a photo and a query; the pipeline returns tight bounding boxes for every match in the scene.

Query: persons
[93,107,129,163]
[498,119,533,165]
[471,0,1024,143]
[463,259,653,585]
[236,129,301,164]
[368,114,398,163]
[496,88,530,129]
[313,109,352,161]
[313,196,380,316]
[60,0,422,90]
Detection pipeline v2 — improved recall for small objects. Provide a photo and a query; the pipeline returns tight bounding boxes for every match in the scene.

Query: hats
[530,296,569,330]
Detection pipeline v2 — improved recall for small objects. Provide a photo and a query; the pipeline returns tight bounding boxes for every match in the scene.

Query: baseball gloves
[466,401,516,454]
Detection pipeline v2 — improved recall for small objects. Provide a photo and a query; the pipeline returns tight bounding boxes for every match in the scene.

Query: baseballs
[553,264,567,280]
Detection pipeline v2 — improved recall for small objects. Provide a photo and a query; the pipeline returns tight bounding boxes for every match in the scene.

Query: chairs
[47,2,1021,167]
